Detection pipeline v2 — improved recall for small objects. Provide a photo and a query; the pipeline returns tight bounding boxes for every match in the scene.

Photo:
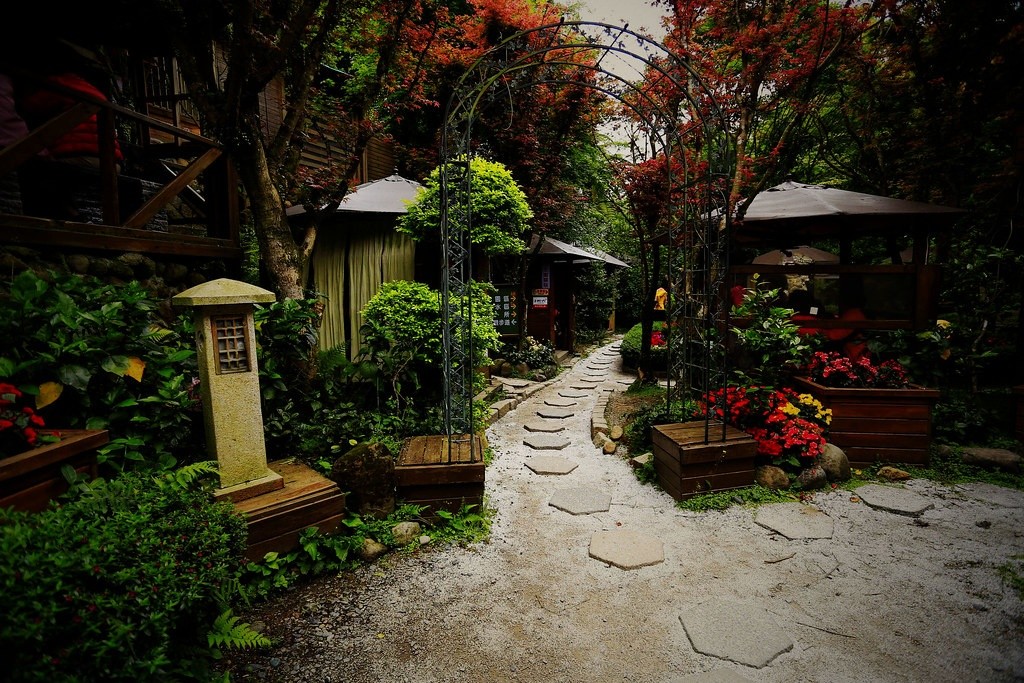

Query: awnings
[554,240,631,269]
[284,174,465,212]
[508,232,604,263]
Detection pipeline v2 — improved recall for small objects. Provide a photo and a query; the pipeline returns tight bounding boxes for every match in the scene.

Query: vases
[794,373,939,470]
[0,427,108,515]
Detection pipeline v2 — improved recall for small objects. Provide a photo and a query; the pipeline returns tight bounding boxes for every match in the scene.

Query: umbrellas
[646,179,974,249]
[733,245,842,267]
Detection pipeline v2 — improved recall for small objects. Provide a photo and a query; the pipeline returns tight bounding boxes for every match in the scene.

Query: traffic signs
[487,283,524,340]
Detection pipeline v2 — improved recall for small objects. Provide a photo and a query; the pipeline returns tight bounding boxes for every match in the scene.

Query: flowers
[1,381,62,453]
[802,349,911,389]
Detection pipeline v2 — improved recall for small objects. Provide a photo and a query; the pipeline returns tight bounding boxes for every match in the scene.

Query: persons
[17,61,124,223]
[756,272,881,320]
[655,277,686,321]
